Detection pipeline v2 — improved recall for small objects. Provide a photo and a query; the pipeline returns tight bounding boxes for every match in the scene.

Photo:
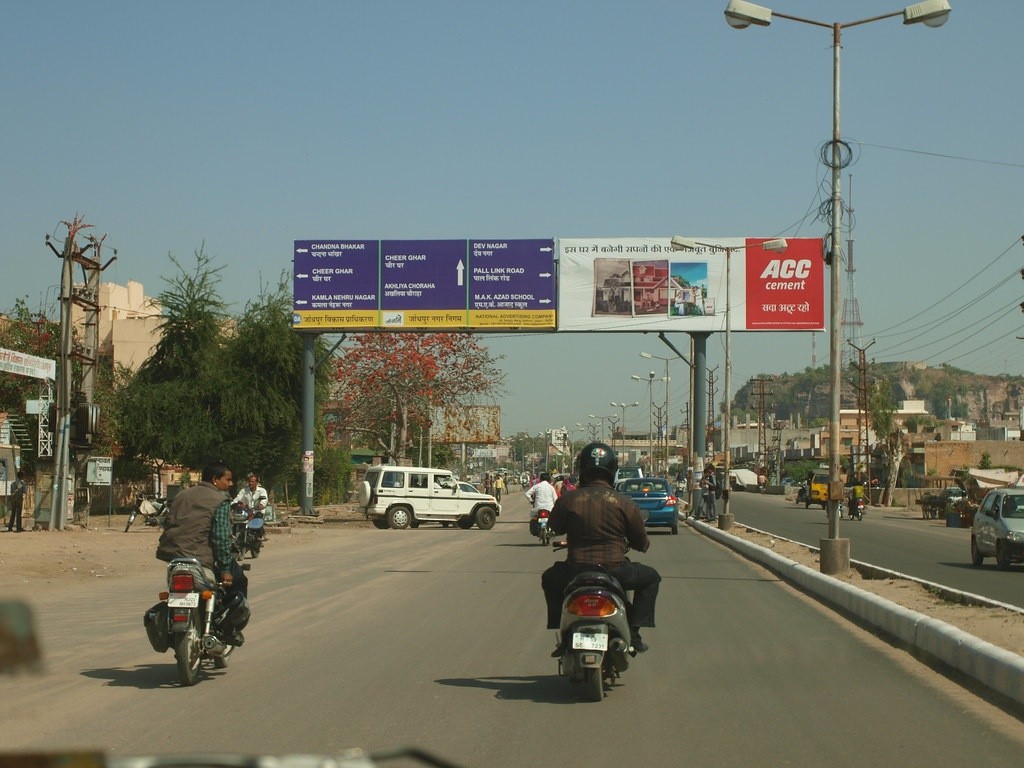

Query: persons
[482,468,867,537]
[155,461,249,647]
[229,475,268,546]
[8,472,26,531]
[540,443,662,656]
[608,286,704,316]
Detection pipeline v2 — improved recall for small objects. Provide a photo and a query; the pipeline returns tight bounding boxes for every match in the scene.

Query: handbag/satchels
[144,601,169,653]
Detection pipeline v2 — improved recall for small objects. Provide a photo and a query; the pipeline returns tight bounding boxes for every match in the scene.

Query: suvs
[358,466,501,530]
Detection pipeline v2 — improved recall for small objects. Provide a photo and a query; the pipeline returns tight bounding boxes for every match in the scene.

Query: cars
[970,488,1024,570]
[943,487,969,504]
[615,467,680,536]
[440,480,480,494]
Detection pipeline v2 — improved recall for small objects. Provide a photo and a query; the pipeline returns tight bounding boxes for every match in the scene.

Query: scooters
[939,503,944,517]
[559,509,653,702]
[796,481,810,504]
[930,506,936,518]
[676,485,685,498]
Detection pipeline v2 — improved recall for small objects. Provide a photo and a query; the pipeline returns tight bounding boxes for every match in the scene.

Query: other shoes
[551,647,561,657]
[631,637,648,652]
[223,632,244,643]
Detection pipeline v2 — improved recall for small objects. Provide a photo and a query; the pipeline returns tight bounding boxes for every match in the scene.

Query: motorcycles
[847,497,863,521]
[805,470,830,509]
[230,496,270,561]
[160,539,253,686]
[533,509,558,547]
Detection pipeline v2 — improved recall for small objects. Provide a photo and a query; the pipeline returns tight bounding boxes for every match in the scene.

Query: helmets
[213,591,250,634]
[579,443,617,485]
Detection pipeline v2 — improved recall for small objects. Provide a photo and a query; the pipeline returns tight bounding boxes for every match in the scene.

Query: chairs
[412,476,428,488]
[631,482,663,492]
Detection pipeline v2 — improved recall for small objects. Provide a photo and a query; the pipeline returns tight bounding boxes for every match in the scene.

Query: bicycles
[921,505,929,519]
[759,484,766,496]
[124,484,173,531]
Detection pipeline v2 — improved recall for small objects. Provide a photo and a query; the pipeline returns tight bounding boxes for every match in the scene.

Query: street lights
[724,0,953,573]
[589,414,616,444]
[610,402,640,465]
[670,235,789,530]
[501,423,601,474]
[640,351,693,478]
[630,375,670,478]
